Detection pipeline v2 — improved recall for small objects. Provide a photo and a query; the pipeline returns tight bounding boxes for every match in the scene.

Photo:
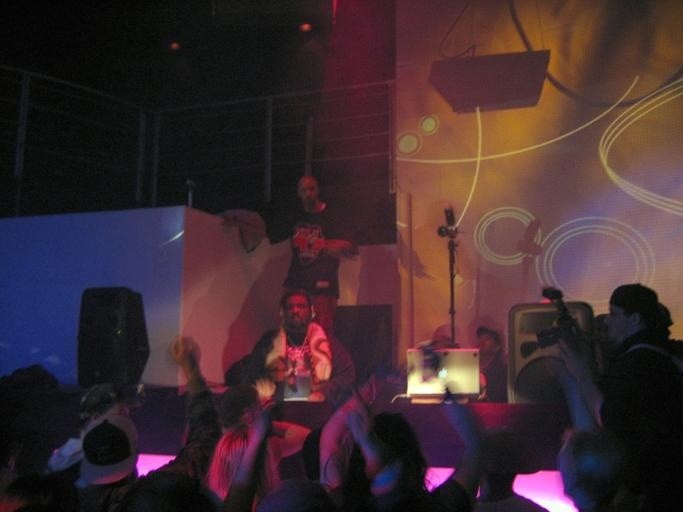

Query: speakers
[78,287,150,405]
[429,49,551,114]
[507,302,594,408]
[335,304,393,387]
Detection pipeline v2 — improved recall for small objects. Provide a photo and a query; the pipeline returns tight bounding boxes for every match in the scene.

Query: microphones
[439,226,458,237]
[443,202,455,226]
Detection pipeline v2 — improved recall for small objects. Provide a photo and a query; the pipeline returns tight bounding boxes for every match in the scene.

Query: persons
[264,174,358,335]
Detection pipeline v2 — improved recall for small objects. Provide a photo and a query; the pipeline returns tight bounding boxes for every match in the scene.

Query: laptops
[406,348,481,399]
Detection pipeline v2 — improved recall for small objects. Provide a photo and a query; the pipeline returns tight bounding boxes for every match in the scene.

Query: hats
[476,326,500,345]
[79,382,138,425]
[79,415,139,486]
[609,282,674,333]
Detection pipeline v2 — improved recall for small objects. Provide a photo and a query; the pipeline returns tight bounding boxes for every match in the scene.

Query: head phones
[279,289,316,321]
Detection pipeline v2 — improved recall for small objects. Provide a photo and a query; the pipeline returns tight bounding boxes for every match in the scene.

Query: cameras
[537,285,586,350]
